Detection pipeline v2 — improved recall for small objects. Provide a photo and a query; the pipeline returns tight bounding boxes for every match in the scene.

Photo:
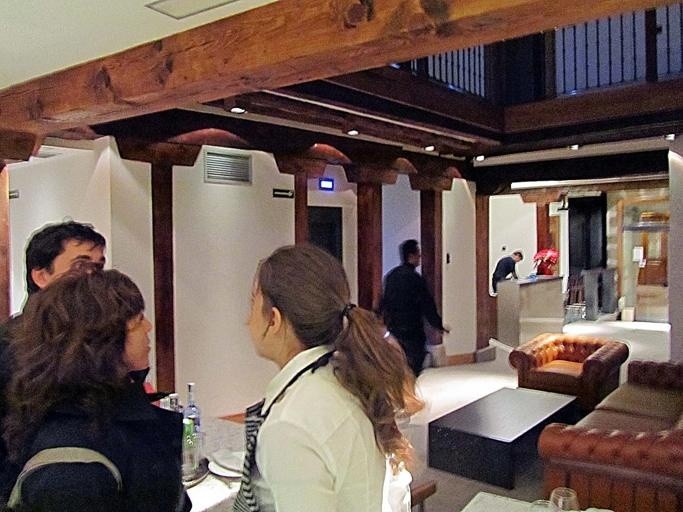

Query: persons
[0,258,193,510]
[491,250,524,294]
[1,217,107,433]
[381,238,450,382]
[226,239,427,510]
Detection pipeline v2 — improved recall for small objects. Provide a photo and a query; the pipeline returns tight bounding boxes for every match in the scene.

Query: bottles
[160,383,201,483]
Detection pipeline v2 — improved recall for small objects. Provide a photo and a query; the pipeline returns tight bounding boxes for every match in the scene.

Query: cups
[528,486,580,512]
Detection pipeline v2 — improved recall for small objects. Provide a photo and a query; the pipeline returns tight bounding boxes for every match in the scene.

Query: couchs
[538,360,682,512]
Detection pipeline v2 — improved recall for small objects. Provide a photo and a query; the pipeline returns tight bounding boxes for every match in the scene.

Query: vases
[538,261,551,274]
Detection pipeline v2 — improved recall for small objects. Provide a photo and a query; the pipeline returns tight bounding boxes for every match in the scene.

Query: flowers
[532,247,559,269]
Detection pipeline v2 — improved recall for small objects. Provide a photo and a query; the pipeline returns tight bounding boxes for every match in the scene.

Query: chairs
[508,333,629,411]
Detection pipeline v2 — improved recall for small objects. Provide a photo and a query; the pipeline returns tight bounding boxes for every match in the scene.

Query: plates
[207,451,247,478]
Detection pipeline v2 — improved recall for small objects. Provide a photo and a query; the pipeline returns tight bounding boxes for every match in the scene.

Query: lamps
[224,93,250,114]
